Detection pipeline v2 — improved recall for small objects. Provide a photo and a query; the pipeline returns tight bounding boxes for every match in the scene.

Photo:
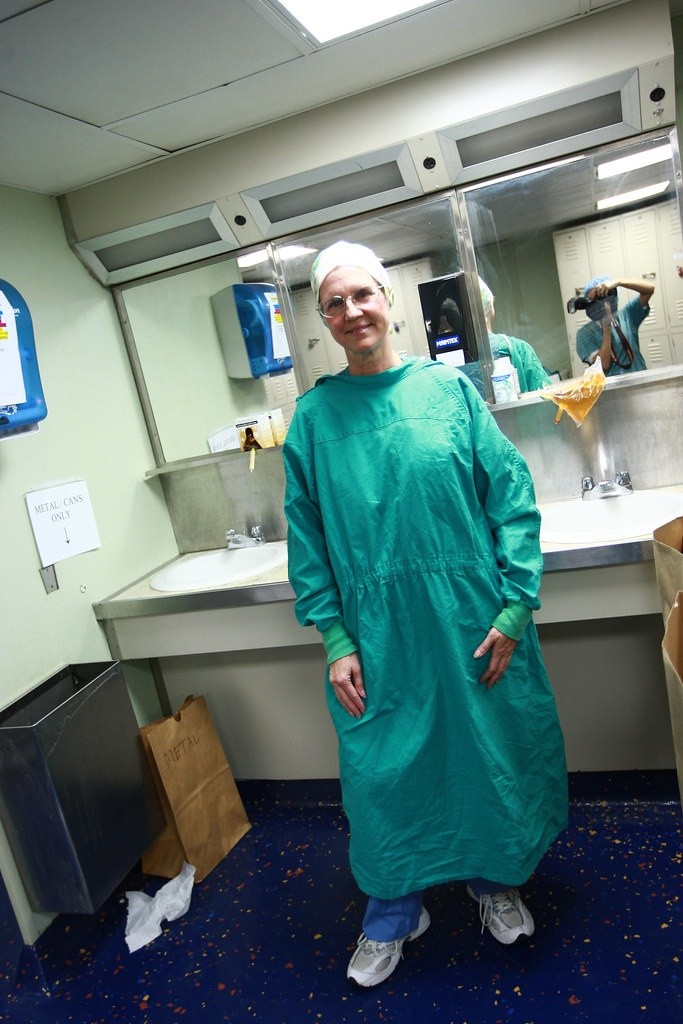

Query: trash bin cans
[0,660,168,915]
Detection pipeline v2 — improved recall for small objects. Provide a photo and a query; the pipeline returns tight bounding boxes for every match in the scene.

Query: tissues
[418,271,479,367]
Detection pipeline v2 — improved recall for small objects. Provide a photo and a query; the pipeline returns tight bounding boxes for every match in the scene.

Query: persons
[573,274,657,377]
[278,239,570,987]
[475,272,551,392]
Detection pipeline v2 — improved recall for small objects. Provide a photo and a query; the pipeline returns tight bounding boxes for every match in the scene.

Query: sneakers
[346,904,430,988]
[467,881,535,945]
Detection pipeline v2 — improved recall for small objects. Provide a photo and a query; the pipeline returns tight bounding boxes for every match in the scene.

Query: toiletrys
[488,360,518,405]
[498,357,521,400]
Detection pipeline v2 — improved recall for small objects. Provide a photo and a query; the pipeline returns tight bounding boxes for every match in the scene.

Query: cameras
[568,293,618,322]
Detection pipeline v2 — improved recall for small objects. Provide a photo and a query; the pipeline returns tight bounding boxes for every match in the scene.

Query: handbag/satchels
[138,696,252,886]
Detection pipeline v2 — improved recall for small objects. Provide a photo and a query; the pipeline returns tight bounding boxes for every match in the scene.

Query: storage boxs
[491,371,519,404]
[250,407,287,445]
[233,413,275,452]
[512,368,520,393]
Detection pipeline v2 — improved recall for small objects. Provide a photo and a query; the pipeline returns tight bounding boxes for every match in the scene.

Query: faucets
[225,524,267,550]
[582,471,634,501]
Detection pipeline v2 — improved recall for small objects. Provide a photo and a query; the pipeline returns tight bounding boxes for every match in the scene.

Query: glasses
[316,281,383,318]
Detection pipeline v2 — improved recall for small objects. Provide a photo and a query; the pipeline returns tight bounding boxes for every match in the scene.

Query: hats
[309,239,393,327]
[478,274,493,316]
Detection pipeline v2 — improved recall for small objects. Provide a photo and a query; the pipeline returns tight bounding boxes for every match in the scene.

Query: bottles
[491,360,518,404]
[501,357,521,395]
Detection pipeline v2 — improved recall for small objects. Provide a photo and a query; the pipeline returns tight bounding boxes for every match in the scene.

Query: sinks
[150,540,288,592]
[536,490,683,543]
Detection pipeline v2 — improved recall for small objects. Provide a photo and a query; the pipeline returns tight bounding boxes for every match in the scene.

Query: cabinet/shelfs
[288,257,449,388]
[552,199,683,378]
[261,367,300,434]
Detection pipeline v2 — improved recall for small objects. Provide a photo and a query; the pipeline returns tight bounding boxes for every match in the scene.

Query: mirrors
[111,124,683,468]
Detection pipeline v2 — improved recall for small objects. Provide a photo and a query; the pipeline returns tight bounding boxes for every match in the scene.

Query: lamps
[593,144,673,179]
[595,181,671,209]
